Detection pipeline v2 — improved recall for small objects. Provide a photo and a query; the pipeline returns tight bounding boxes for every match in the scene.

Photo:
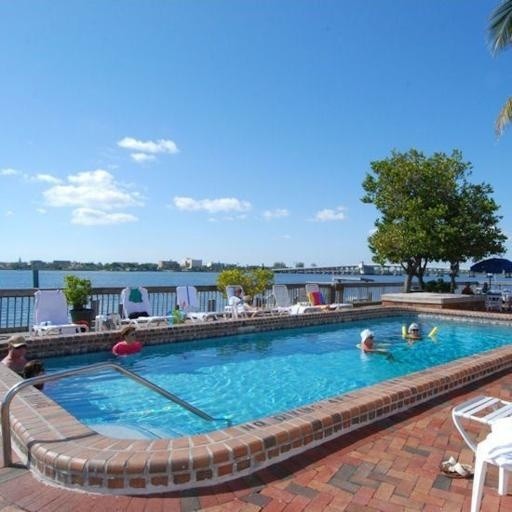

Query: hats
[9,335,26,347]
[361,329,374,343]
[408,324,419,333]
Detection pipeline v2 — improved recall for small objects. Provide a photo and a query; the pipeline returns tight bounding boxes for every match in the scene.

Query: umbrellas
[471,258,512,290]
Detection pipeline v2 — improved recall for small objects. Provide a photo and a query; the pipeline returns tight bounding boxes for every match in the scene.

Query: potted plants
[63,274,94,333]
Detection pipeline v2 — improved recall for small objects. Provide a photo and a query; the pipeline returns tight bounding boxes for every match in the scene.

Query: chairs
[450,394,512,512]
[304,283,352,310]
[176,286,231,322]
[33,287,90,335]
[474,288,512,311]
[223,285,269,319]
[267,285,322,317]
[119,285,177,329]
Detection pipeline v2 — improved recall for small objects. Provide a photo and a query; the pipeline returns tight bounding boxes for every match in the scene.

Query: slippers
[439,457,473,478]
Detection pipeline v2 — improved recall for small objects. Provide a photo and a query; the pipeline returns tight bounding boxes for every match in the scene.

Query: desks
[95,313,119,332]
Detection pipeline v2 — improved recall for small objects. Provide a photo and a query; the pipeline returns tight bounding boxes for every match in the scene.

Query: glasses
[367,336,373,339]
[128,333,137,337]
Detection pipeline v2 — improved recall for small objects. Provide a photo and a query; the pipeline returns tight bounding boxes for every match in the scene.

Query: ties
[411,328,419,331]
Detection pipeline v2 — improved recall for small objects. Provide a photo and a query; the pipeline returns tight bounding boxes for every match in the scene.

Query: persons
[462,283,475,295]
[20,359,45,391]
[115,326,142,355]
[408,322,420,345]
[482,281,490,293]
[1,334,29,378]
[360,328,376,354]
[228,286,261,311]
